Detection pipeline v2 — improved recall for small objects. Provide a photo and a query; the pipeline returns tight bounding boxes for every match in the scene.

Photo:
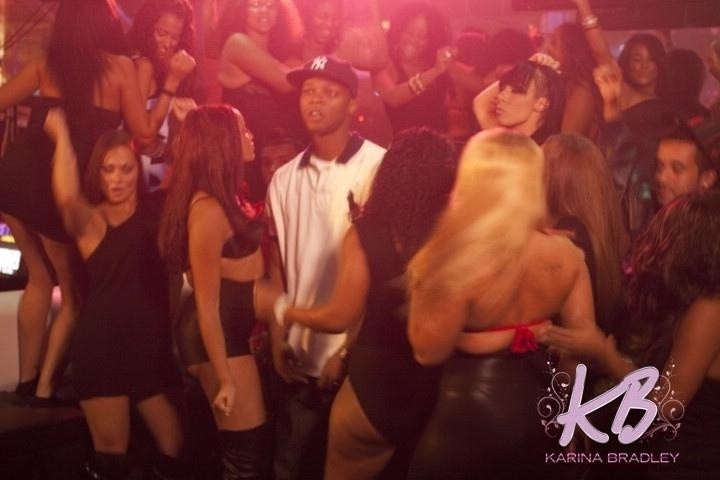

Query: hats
[286,56,358,97]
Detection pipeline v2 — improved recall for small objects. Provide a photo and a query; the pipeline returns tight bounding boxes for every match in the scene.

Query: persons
[246,128,306,201]
[539,131,624,404]
[251,126,459,480]
[156,102,269,480]
[472,52,567,148]
[280,0,344,67]
[592,48,713,201]
[374,4,519,142]
[262,55,386,480]
[123,0,195,206]
[43,103,186,480]
[537,188,720,480]
[640,114,720,209]
[572,0,668,113]
[526,20,597,138]
[214,0,345,142]
[705,35,720,116]
[0,0,61,291]
[0,0,197,409]
[403,124,595,480]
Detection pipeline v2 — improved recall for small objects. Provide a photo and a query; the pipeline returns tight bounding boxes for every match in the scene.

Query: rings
[445,52,452,58]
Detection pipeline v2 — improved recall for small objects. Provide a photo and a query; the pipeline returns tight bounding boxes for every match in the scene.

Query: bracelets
[336,344,351,365]
[581,14,600,31]
[160,87,175,98]
[407,73,427,97]
[272,294,288,327]
